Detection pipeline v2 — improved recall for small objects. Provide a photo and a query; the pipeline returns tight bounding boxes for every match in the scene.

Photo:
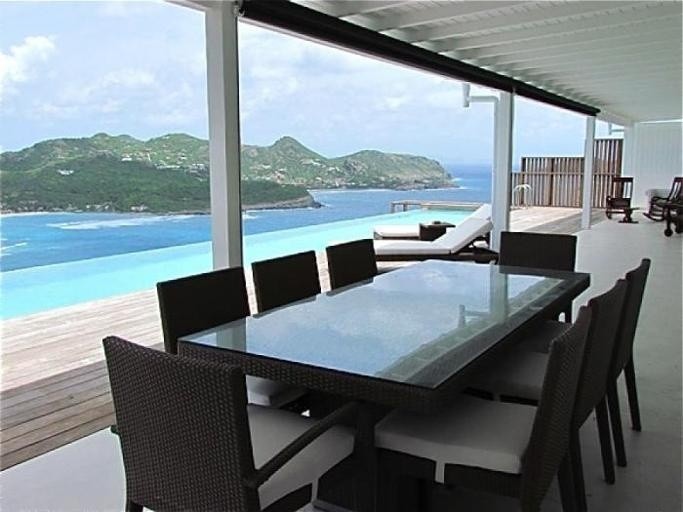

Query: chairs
[641,175,683,237]
[605,174,635,219]
